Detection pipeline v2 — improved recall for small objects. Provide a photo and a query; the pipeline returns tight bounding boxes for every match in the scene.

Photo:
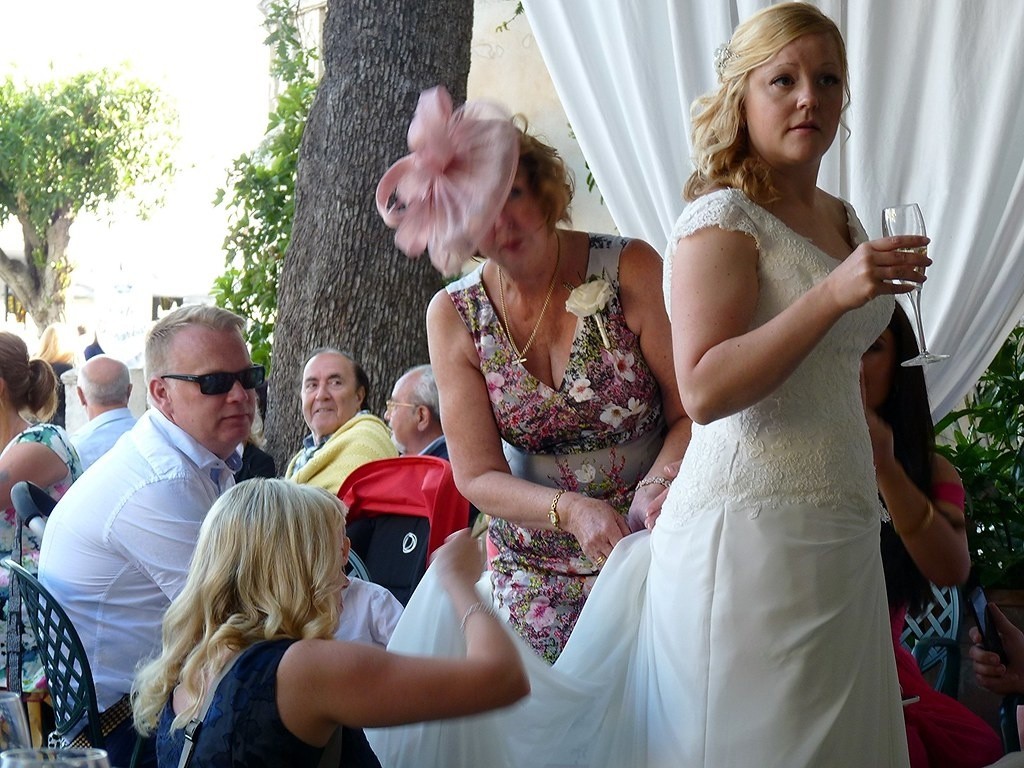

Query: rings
[595,556,606,567]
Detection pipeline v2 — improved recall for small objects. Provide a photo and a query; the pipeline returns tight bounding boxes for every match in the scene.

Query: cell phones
[970,586,1008,678]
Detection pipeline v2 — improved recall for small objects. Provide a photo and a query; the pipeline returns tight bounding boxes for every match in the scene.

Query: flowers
[565,275,614,349]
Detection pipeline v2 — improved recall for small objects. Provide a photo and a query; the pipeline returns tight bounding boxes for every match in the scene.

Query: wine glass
[881,203,950,367]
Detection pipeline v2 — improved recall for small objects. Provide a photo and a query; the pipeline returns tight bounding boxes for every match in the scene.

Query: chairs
[1,558,147,768]
[899,578,1024,755]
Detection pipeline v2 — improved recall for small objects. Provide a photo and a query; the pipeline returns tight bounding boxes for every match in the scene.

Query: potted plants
[931,326,1024,632]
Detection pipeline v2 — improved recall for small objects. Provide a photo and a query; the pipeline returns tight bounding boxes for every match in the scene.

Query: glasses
[161,362,267,397]
[385,399,422,416]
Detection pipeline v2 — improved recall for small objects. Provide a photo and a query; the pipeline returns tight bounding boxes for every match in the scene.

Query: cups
[0,749,111,768]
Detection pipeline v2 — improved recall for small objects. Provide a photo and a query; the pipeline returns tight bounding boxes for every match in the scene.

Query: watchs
[548,489,568,533]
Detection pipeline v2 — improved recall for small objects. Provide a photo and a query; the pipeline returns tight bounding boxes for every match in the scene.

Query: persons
[969,601,1024,768]
[37,304,257,768]
[619,4,933,768]
[426,113,691,665]
[300,485,404,768]
[860,298,1002,768]
[0,322,140,702]
[130,478,531,768]
[226,348,481,527]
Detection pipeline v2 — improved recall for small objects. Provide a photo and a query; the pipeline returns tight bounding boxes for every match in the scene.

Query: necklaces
[496,228,564,365]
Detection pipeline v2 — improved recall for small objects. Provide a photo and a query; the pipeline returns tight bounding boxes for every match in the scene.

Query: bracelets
[890,497,935,540]
[636,476,670,488]
[460,603,494,629]
